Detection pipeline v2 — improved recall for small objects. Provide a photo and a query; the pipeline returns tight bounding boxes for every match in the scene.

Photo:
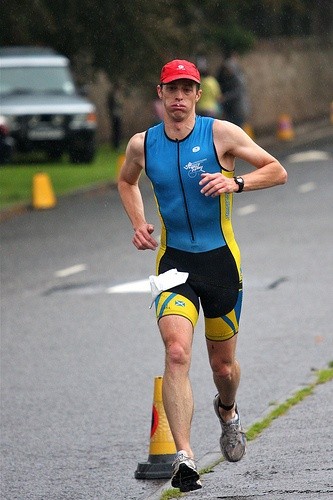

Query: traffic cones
[29,172,57,210]
[133,375,179,481]
[278,114,297,141]
[109,155,130,186]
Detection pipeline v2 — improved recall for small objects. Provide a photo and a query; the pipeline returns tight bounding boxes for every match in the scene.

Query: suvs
[0,46,100,164]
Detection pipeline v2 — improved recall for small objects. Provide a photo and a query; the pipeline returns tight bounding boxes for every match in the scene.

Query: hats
[160,59,202,86]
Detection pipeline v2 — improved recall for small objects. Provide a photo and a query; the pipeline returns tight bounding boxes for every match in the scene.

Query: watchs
[233,177,244,193]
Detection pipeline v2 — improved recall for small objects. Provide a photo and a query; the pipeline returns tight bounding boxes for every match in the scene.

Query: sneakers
[213,393,245,463]
[169,451,203,492]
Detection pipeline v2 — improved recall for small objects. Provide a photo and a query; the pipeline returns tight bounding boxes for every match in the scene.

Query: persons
[117,60,287,494]
[75,77,87,97]
[216,55,254,139]
[195,65,225,121]
[108,78,122,154]
[150,90,163,126]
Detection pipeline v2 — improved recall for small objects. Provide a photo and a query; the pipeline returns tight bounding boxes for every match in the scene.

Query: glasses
[164,84,193,95]
